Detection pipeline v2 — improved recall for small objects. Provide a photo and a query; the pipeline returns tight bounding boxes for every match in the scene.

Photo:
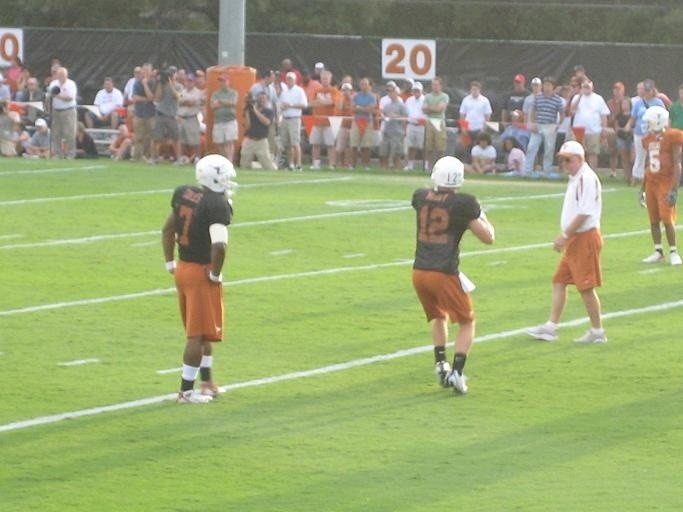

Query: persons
[159,150,240,408]
[638,104,683,267]
[525,138,614,347]
[241,58,449,171]
[410,152,497,395]
[459,65,683,206]
[84,62,238,163]
[1,55,97,159]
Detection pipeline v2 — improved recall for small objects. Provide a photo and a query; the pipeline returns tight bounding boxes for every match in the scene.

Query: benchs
[24,125,120,158]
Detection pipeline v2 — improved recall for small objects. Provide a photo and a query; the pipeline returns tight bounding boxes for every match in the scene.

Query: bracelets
[208,270,223,283]
[560,231,568,241]
[164,260,176,271]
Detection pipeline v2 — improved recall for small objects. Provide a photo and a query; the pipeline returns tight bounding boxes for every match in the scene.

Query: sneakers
[435,361,452,388]
[287,159,430,171]
[523,325,560,341]
[670,252,682,266]
[114,152,199,166]
[444,369,469,395]
[642,251,665,263]
[178,389,213,404]
[573,328,608,344]
[201,381,226,395]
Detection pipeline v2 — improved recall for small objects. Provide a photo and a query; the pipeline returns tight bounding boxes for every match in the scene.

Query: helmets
[641,106,669,133]
[196,154,238,199]
[431,155,466,188]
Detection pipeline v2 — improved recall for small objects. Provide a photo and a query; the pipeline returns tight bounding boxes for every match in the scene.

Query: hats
[531,77,542,85]
[286,72,297,80]
[216,73,231,83]
[555,140,585,158]
[581,79,593,87]
[514,74,525,82]
[315,62,325,69]
[341,83,353,90]
[613,82,625,89]
[8,112,21,124]
[509,109,522,120]
[185,74,196,81]
[34,119,47,128]
[386,77,423,95]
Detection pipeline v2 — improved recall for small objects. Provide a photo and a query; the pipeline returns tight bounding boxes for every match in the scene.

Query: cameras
[47,86,60,95]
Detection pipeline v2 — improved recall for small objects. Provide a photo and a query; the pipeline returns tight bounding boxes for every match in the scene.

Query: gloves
[665,188,678,207]
[638,190,645,207]
